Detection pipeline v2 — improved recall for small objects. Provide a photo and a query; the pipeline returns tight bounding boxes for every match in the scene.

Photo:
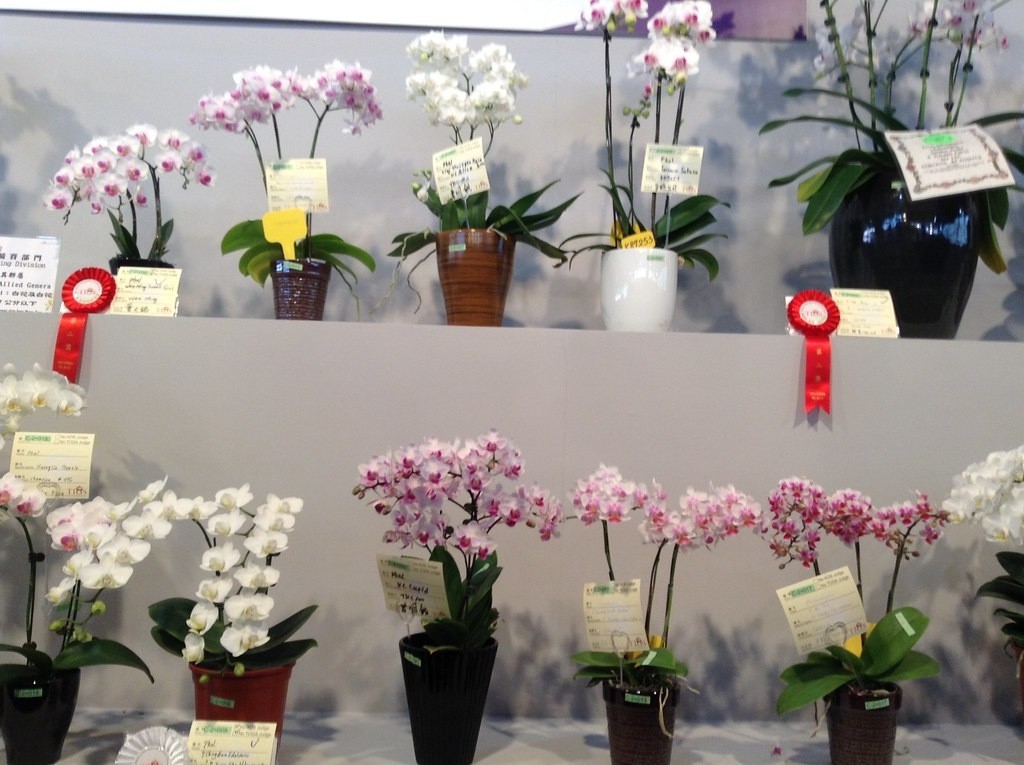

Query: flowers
[148,483,318,683]
[188,60,383,317]
[943,444,1024,657]
[558,0,731,283]
[0,472,169,685]
[758,0,1023,275]
[45,122,214,260]
[352,431,565,653]
[569,464,769,688]
[368,31,577,318]
[0,363,87,450]
[753,477,947,737]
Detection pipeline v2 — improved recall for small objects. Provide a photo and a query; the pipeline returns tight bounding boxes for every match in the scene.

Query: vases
[823,682,902,765]
[1010,640,1024,715]
[0,667,82,765]
[187,661,296,749]
[398,632,498,765]
[109,258,175,276]
[270,171,979,339]
[602,680,680,765]
